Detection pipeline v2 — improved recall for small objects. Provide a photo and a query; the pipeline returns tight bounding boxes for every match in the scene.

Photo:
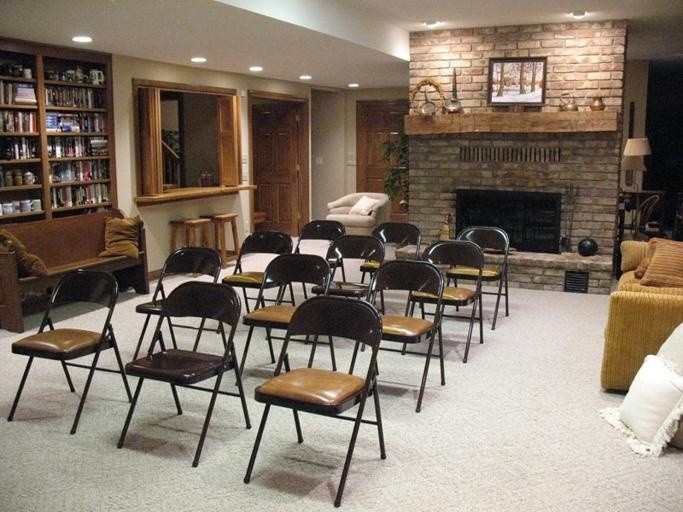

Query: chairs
[323,191,393,236]
[618,194,658,242]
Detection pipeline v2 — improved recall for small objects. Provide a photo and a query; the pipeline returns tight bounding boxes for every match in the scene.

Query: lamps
[618,153,646,192]
[622,136,652,191]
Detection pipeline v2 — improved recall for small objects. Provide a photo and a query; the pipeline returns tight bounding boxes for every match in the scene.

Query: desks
[616,189,665,280]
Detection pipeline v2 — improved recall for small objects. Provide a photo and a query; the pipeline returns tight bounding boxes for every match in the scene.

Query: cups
[46,67,104,87]
[0,199,40,216]
[22,68,31,79]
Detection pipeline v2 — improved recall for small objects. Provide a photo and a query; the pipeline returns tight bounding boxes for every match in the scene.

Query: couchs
[1,209,150,333]
[601,236,683,397]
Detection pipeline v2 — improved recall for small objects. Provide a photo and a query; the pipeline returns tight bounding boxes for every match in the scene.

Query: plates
[419,102,435,114]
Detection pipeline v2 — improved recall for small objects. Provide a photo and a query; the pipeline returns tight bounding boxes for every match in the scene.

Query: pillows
[98,215,144,259]
[650,322,683,450]
[615,351,682,449]
[1,229,49,277]
[348,194,382,216]
[631,237,682,277]
[639,236,683,287]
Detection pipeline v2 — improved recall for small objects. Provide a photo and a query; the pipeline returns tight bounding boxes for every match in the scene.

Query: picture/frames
[485,56,548,107]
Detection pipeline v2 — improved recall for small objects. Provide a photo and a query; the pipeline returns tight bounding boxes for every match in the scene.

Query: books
[1,81,110,208]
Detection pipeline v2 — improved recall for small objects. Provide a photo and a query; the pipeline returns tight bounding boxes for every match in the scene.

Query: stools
[200,213,241,267]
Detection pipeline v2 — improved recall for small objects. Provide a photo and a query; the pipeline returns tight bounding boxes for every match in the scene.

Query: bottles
[589,97,605,110]
[0,168,35,187]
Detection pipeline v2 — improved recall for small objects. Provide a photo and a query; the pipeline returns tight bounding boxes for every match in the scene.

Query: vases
[590,96,604,111]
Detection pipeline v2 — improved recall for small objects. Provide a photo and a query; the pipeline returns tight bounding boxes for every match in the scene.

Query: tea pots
[443,98,461,113]
[559,92,578,112]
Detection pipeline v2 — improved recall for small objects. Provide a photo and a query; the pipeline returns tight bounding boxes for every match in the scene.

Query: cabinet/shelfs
[0,37,114,223]
